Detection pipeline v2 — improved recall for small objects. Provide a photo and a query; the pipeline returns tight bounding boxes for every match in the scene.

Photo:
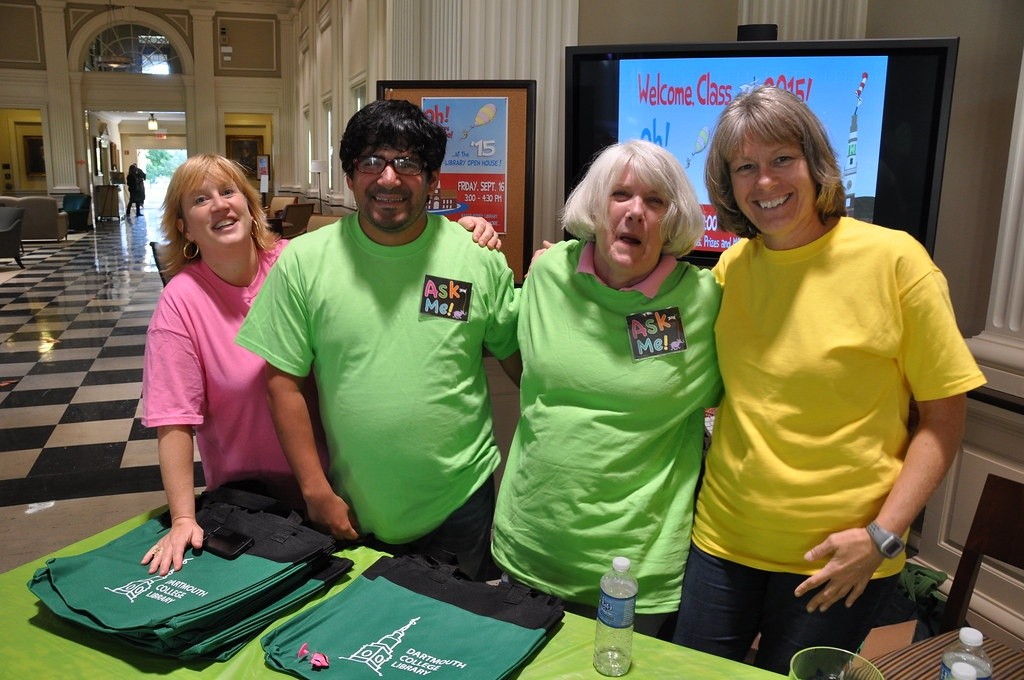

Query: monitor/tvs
[564,37,962,266]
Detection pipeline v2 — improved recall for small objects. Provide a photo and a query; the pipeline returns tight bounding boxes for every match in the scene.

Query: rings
[152,548,163,556]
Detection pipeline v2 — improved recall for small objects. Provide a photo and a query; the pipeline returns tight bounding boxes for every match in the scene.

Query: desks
[1,496,793,680]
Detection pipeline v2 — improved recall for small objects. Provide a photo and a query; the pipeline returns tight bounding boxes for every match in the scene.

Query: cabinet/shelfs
[94,185,120,222]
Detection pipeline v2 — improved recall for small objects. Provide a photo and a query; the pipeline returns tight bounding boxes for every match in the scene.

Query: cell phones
[200,527,254,560]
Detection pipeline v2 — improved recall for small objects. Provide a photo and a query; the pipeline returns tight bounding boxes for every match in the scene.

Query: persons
[141,152,502,576]
[524,88,989,680]
[481,139,725,646]
[230,98,524,584]
[126,163,147,215]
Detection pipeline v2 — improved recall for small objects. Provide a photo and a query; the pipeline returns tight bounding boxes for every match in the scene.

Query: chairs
[280,202,315,238]
[306,215,341,233]
[270,196,298,218]
[847,472,1024,680]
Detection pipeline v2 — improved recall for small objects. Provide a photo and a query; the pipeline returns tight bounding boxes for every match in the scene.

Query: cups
[593,557,638,676]
[788,648,885,680]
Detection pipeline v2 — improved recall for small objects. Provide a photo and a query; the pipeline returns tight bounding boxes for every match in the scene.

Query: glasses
[353,154,426,176]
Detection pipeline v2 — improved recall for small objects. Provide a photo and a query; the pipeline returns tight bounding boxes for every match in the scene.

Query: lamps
[310,160,327,214]
[260,173,269,206]
[147,114,167,139]
[93,0,133,67]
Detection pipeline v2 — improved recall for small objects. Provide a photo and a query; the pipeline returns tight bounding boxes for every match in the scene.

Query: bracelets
[172,515,194,520]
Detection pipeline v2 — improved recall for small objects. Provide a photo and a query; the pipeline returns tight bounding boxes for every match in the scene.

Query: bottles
[939,627,993,680]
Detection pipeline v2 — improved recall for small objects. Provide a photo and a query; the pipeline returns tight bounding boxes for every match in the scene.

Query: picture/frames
[376,79,536,288]
[225,134,263,177]
[256,154,270,180]
[22,135,45,176]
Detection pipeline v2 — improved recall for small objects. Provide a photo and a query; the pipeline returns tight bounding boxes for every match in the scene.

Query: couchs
[57,193,92,233]
[0,207,26,269]
[0,196,69,243]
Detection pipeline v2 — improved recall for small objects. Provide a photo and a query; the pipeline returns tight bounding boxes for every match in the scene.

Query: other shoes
[126,211,130,214]
[136,213,143,216]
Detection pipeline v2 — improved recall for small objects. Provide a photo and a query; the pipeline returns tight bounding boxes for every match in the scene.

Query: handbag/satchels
[258,551,567,680]
[26,480,360,664]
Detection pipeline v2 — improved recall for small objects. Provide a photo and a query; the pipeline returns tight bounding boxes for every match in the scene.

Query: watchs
[865,519,904,559]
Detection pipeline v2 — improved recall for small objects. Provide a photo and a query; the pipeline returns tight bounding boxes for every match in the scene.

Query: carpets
[21,231,88,247]
[0,266,28,285]
